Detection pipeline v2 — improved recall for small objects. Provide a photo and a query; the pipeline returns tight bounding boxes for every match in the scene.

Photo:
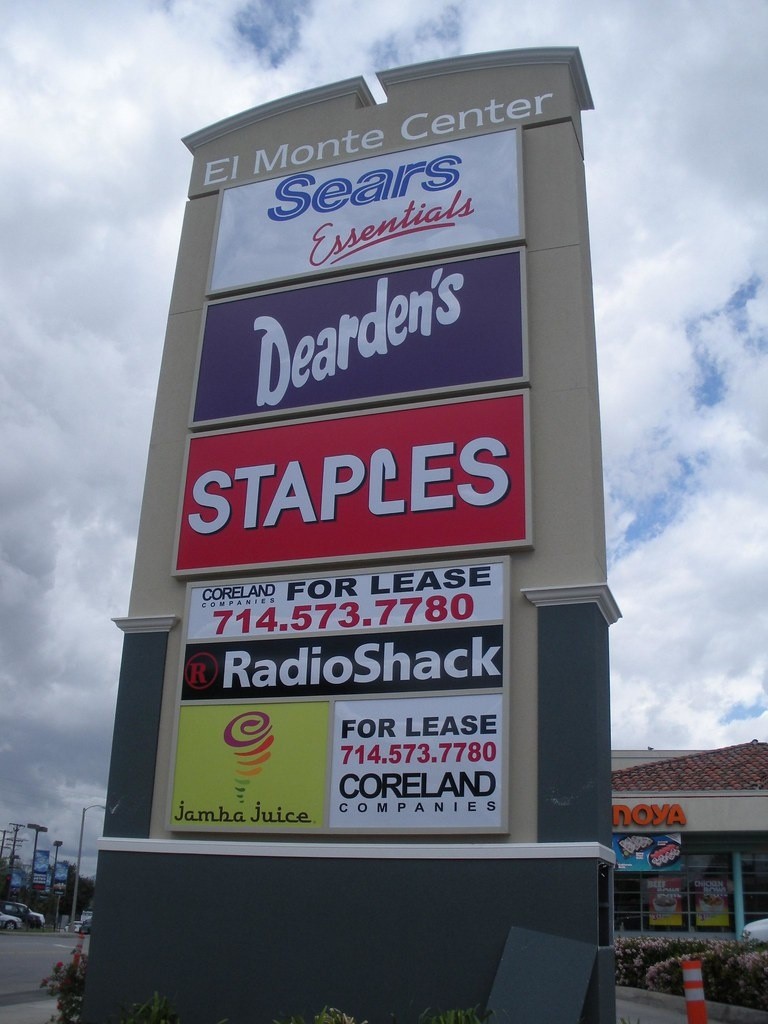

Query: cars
[0,910,22,929]
[742,916,768,945]
[69,911,94,934]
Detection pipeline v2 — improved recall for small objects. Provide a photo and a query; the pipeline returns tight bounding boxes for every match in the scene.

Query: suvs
[0,900,45,929]
